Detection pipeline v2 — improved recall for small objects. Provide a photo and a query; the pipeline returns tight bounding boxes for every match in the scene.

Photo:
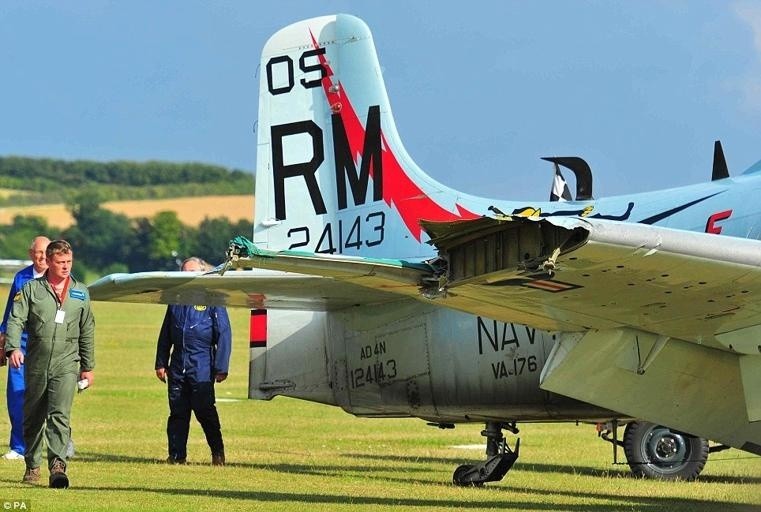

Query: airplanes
[86,13,761,485]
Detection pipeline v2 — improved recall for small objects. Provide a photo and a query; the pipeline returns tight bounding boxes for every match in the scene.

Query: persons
[4,240,96,489]
[0,236,75,461]
[154,256,232,466]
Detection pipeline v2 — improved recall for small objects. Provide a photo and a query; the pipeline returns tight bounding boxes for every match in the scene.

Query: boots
[49,458,69,488]
[23,467,40,481]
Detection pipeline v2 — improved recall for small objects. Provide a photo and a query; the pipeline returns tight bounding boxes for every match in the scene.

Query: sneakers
[212,455,225,466]
[167,456,186,463]
[2,450,24,459]
